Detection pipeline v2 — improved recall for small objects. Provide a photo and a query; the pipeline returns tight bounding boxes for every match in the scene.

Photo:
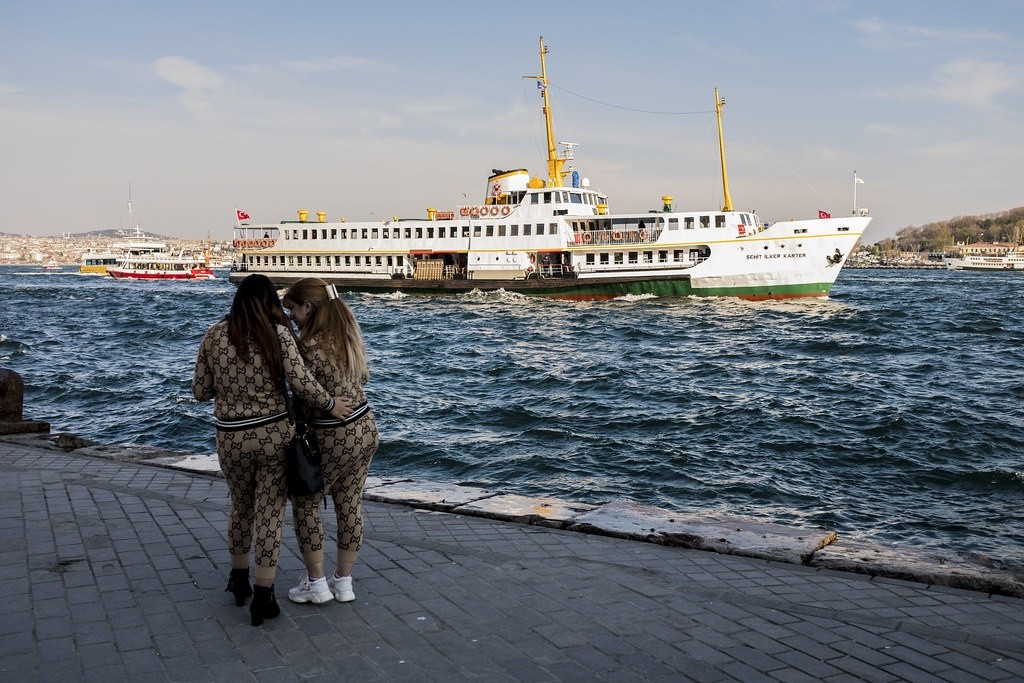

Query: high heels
[225,566,253,605]
[250,584,280,626]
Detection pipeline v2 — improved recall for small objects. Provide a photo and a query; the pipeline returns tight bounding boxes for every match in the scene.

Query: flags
[237,210,250,220]
[855,177,864,184]
[537,81,547,90]
[819,210,831,219]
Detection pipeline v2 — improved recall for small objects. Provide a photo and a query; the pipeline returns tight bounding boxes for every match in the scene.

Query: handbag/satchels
[285,430,324,500]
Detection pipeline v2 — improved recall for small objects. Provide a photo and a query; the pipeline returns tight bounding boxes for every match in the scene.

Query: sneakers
[326,570,355,602]
[288,573,334,603]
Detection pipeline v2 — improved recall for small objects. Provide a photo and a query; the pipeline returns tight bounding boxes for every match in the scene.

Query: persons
[264,231,269,238]
[604,222,611,230]
[638,220,646,242]
[191,273,354,626]
[283,277,380,603]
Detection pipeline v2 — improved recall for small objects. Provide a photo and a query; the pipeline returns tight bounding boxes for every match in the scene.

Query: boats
[41,260,63,269]
[942,251,1024,271]
[78,199,224,280]
[228,35,875,297]
